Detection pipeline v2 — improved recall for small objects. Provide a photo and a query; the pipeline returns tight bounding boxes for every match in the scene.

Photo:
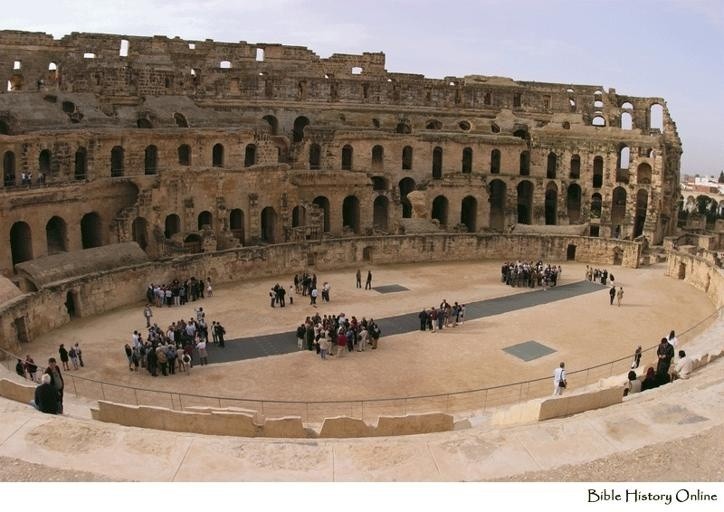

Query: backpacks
[183,356,189,362]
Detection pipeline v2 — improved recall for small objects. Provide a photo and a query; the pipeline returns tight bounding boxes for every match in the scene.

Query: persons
[16,342,85,415]
[616,287,624,308]
[501,260,561,291]
[419,299,466,333]
[609,286,616,305]
[553,362,567,395]
[624,330,693,397]
[585,264,615,287]
[269,270,381,360]
[125,276,226,377]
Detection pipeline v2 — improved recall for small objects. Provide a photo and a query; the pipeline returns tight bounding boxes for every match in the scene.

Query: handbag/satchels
[559,381,565,387]
